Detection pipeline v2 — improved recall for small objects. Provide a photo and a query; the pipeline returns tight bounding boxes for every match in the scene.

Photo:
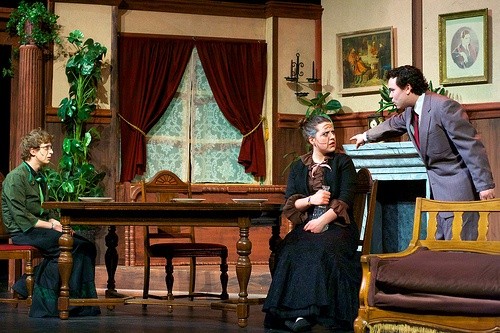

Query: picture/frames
[335,26,395,95]
[437,8,489,85]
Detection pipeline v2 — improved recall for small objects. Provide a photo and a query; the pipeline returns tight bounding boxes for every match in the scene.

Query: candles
[291,59,293,78]
[312,61,314,79]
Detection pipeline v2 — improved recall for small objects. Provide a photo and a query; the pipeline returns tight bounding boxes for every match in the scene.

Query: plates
[171,199,207,203]
[232,198,268,203]
[78,197,112,201]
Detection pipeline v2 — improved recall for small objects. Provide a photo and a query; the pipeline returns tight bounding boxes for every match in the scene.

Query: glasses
[40,145,53,150]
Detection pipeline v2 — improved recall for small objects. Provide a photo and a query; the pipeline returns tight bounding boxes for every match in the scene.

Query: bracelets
[308,196,312,206]
[52,222,54,228]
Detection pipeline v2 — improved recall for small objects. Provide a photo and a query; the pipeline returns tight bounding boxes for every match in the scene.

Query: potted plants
[2,1,68,78]
[41,37,107,244]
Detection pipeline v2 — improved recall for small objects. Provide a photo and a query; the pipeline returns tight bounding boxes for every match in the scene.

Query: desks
[43,201,284,328]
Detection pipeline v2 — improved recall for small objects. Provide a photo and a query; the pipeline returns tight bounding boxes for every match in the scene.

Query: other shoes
[293,318,314,333]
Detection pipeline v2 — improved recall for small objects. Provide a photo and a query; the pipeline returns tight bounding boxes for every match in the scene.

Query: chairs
[347,167,378,254]
[0,173,45,317]
[140,170,229,313]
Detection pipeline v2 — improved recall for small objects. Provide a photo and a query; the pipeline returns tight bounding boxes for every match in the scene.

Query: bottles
[312,186,331,232]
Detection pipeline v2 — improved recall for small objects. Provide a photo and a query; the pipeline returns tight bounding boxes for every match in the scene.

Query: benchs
[353,197,500,333]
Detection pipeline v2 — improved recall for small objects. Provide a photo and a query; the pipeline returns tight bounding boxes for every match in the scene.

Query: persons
[2,127,101,318]
[262,115,362,332]
[349,65,495,241]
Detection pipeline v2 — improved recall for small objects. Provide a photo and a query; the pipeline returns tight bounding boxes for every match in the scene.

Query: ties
[411,108,420,151]
